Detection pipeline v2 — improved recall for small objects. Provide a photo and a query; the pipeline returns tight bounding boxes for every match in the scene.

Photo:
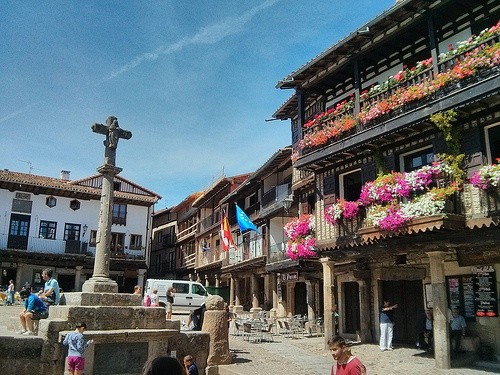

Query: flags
[220,209,237,251]
[236,203,261,236]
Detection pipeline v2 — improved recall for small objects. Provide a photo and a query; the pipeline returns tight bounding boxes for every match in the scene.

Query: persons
[5,280,64,307]
[19,288,49,336]
[142,356,184,375]
[326,335,366,375]
[108,119,122,148]
[184,300,232,331]
[424,304,467,360]
[166,287,175,320]
[37,268,60,306]
[62,321,94,375]
[151,287,159,307]
[146,294,151,307]
[378,298,398,351]
[132,285,142,294]
[183,354,199,375]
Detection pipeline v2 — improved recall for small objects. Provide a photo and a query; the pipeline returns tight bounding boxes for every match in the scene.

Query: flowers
[468,158,500,194]
[323,153,467,233]
[283,213,319,261]
[291,21,500,161]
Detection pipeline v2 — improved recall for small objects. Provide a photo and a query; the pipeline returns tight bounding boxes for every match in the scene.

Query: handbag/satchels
[47,291,56,302]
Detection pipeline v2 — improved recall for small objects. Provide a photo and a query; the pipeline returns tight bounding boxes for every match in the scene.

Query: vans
[144,278,210,307]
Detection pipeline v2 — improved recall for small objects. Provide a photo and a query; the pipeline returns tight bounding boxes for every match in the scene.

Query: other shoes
[16,328,35,336]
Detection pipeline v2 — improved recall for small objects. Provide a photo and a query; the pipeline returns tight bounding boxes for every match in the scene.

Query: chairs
[234,311,325,343]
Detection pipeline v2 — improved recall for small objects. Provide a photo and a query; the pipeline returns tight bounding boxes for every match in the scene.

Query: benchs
[416,335,480,352]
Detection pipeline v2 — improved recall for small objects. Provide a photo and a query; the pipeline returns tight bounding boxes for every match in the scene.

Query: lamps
[395,253,406,267]
[83,224,88,237]
